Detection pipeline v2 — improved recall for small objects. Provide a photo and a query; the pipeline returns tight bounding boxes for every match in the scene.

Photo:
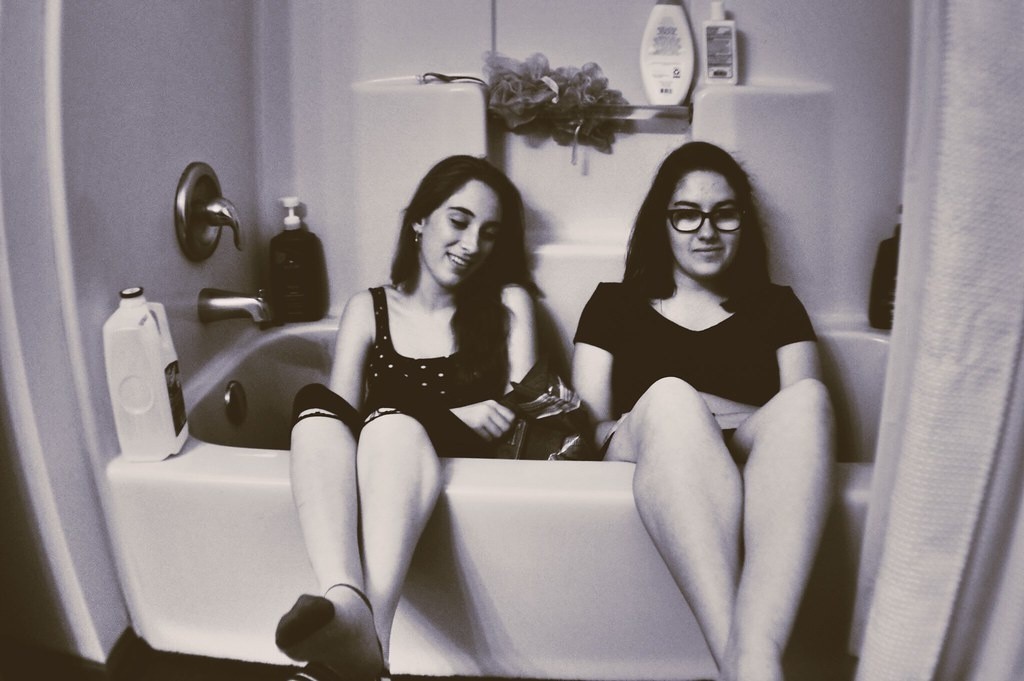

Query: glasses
[664,209,741,233]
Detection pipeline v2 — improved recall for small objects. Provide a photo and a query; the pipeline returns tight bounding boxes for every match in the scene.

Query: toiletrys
[641,0,696,108]
[867,203,906,331]
[266,198,329,326]
[701,2,742,89]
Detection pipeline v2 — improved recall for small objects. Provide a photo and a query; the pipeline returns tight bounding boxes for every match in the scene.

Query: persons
[274,153,544,681]
[573,139,839,678]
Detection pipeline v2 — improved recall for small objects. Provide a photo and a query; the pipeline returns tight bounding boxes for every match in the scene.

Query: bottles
[102,286,188,463]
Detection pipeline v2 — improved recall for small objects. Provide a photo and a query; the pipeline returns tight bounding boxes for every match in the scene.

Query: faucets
[196,287,276,333]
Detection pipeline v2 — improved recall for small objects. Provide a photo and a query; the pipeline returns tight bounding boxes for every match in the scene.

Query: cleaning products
[100,285,190,460]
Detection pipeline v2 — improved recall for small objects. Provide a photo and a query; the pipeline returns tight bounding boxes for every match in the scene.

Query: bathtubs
[98,309,890,679]
